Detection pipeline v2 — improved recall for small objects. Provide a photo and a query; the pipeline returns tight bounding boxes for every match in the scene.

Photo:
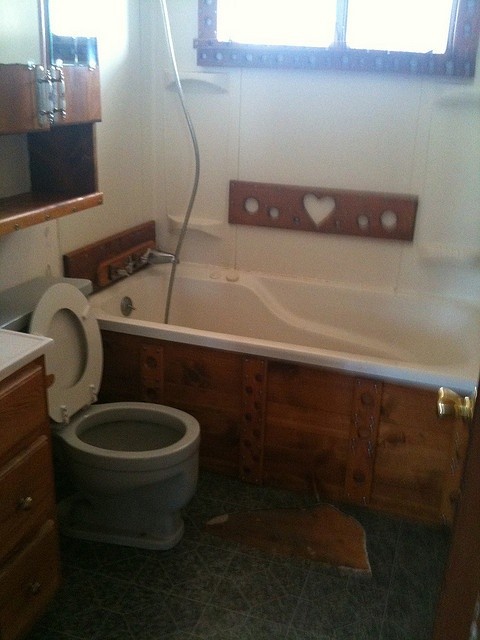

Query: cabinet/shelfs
[0,326,66,639]
[0,62,104,239]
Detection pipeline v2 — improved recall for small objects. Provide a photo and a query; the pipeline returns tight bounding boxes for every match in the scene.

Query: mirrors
[47,0,132,87]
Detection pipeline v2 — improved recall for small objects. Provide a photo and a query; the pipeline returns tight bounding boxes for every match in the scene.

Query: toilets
[29,282,202,551]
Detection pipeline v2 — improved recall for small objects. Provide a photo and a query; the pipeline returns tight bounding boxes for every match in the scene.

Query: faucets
[135,247,179,269]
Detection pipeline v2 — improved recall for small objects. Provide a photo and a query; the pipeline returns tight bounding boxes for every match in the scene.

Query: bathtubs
[86,259,479,392]
